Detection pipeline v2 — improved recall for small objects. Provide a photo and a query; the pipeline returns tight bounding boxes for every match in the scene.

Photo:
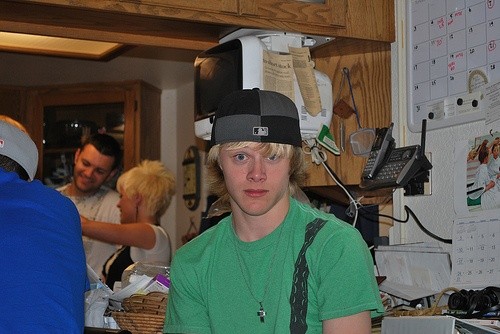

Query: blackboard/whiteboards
[405,0,499,133]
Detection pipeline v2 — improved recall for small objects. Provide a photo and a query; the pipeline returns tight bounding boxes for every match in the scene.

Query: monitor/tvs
[193,35,334,143]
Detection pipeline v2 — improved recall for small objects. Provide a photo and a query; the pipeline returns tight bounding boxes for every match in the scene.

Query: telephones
[359,122,433,189]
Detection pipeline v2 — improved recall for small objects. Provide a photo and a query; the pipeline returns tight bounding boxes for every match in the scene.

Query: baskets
[112,291,167,334]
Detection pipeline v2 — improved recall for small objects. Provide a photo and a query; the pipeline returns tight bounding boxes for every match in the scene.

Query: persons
[0,111,91,334]
[475,138,500,208]
[81,159,171,294]
[55,133,121,287]
[161,88,385,334]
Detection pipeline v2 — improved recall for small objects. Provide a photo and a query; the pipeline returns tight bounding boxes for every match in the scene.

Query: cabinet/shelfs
[26,79,162,186]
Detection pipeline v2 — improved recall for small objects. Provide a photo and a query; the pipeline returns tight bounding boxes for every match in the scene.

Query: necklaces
[230,208,286,323]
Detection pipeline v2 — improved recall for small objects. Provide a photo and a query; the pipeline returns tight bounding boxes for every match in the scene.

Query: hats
[210,87,303,148]
[0,115,39,182]
